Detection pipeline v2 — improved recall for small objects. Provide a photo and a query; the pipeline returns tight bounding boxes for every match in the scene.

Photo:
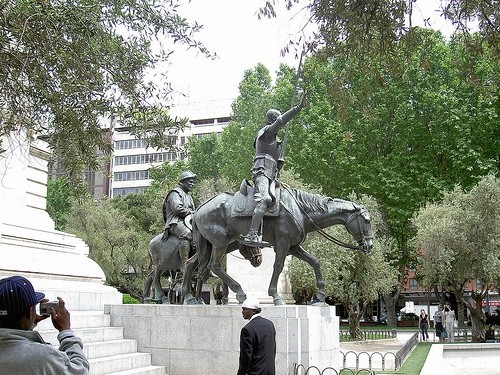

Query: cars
[384,312,434,328]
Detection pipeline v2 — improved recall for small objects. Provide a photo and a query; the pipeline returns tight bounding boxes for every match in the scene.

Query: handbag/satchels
[441,328,448,338]
[453,317,458,328]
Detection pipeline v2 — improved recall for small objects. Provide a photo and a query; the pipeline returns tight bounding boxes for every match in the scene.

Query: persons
[237,297,277,375]
[243,91,307,248]
[162,169,200,278]
[0,275,89,375]
[418,309,431,342]
[434,303,447,343]
[440,305,456,344]
[484,324,500,343]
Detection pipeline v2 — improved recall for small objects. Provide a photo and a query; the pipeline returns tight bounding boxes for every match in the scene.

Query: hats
[241,297,260,309]
[0,276,45,315]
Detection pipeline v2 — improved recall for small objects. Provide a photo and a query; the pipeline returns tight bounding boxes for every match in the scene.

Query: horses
[143,188,373,307]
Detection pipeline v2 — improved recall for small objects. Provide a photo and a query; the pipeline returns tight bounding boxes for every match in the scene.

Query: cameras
[40,301,59,315]
